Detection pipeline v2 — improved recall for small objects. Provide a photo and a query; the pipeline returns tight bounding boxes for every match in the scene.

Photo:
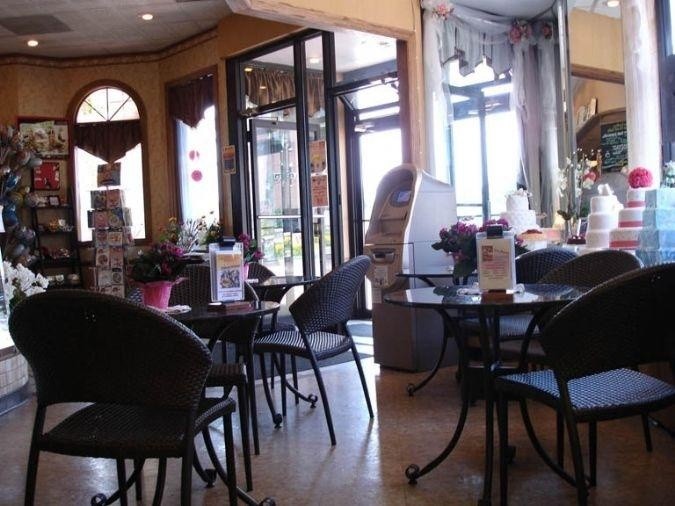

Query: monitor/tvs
[396,190,412,202]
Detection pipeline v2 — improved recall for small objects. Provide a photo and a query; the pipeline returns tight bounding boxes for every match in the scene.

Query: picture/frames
[586,96,598,121]
[575,104,586,128]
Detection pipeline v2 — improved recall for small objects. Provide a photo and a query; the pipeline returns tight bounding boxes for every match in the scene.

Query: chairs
[125,269,260,463]
[497,260,675,500]
[442,249,645,478]
[452,246,581,396]
[245,255,374,447]
[7,289,236,506]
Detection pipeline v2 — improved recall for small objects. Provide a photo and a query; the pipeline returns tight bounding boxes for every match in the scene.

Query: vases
[134,279,178,309]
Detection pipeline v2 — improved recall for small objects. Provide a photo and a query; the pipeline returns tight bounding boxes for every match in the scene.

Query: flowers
[432,216,529,287]
[554,145,602,239]
[161,215,267,265]
[0,122,52,298]
[505,15,552,45]
[421,0,454,23]
[122,242,195,281]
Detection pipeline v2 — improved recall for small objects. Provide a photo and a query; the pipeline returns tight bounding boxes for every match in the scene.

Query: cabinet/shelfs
[19,186,84,290]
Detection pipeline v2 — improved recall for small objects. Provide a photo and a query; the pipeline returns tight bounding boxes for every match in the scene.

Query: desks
[382,284,593,503]
[244,275,322,389]
[397,265,508,405]
[121,299,280,499]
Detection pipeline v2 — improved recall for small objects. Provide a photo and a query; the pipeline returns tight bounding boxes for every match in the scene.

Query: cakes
[636,159,675,266]
[608,166,652,249]
[500,188,548,252]
[585,183,624,248]
